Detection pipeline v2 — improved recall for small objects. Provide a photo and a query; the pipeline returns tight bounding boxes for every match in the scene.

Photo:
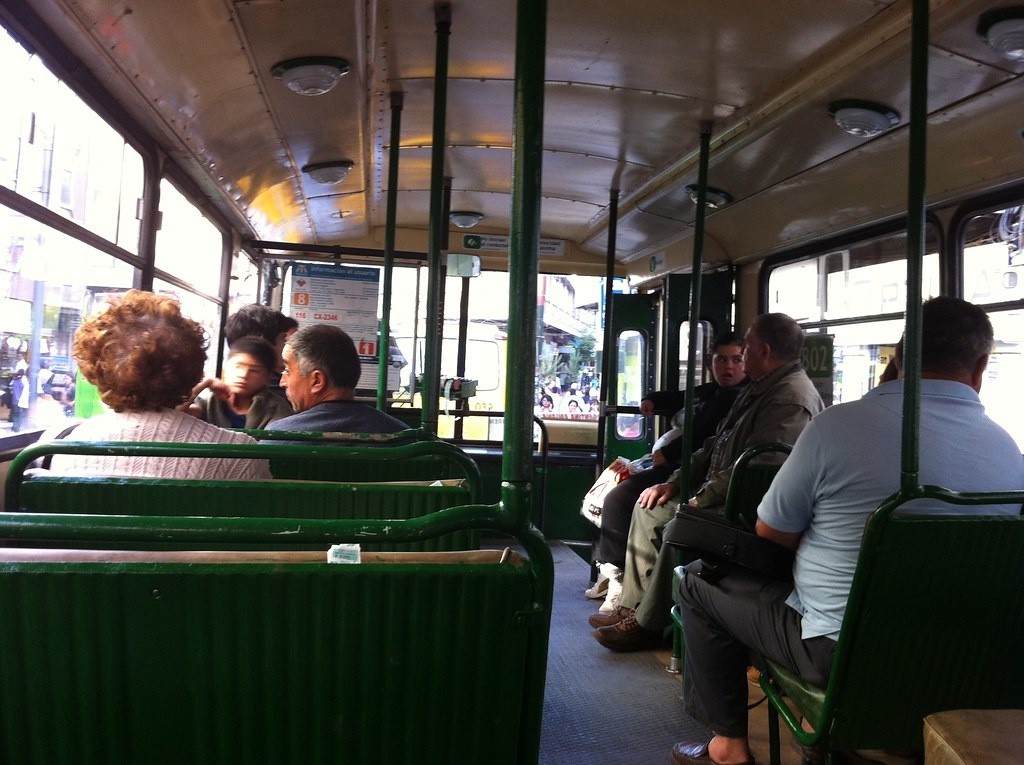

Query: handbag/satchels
[579,454,658,530]
[664,503,795,586]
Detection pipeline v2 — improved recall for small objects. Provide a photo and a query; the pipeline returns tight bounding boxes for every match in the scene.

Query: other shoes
[599,563,624,614]
[585,570,610,598]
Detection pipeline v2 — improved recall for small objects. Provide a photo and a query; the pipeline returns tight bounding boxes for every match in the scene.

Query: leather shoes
[670,740,755,765]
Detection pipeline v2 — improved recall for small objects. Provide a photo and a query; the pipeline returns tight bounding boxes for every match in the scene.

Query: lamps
[826,99,900,137]
[449,211,484,228]
[301,159,353,185]
[975,5,1024,62]
[686,185,733,208]
[272,52,350,96]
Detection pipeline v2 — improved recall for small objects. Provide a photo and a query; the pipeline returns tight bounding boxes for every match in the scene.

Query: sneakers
[594,608,665,653]
[588,608,635,628]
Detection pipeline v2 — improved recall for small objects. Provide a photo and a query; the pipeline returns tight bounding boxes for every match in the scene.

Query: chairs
[758,481,1024,765]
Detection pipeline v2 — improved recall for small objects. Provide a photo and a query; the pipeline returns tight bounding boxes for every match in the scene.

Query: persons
[583,332,753,615]
[223,301,300,410]
[258,324,419,449]
[61,373,76,417]
[587,312,826,652]
[32,288,271,480]
[7,354,55,432]
[668,296,1024,765]
[174,335,294,430]
[534,374,599,414]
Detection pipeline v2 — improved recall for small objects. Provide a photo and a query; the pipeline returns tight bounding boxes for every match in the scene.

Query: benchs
[0,424,554,765]
